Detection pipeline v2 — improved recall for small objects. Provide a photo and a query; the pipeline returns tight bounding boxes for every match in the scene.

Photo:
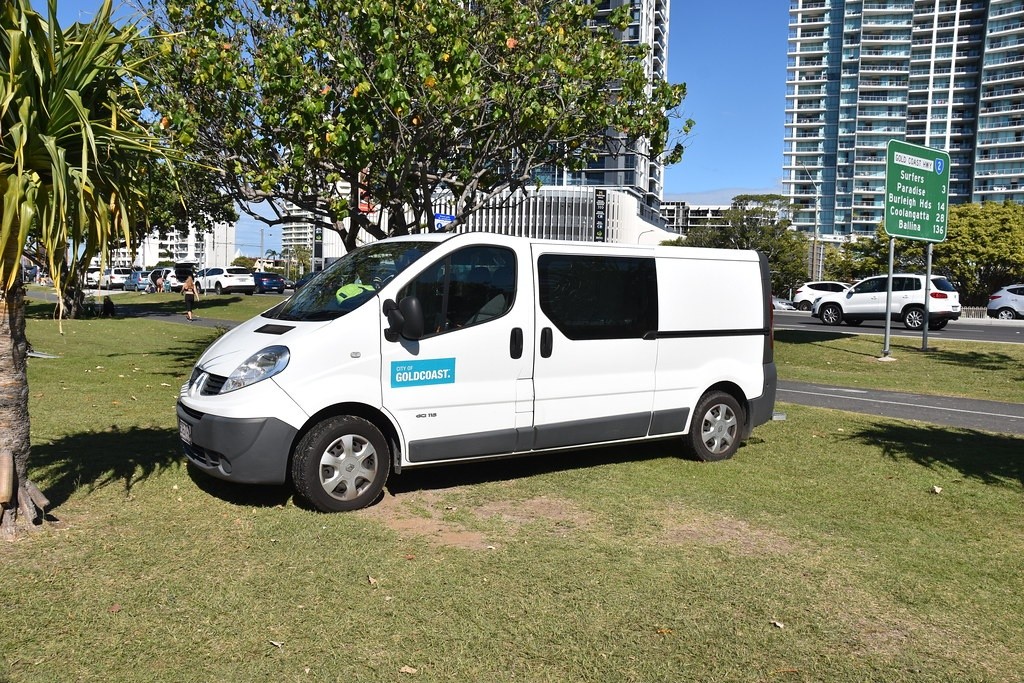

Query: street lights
[796,160,819,281]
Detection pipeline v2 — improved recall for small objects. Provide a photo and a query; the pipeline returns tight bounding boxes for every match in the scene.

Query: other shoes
[186,318,192,322]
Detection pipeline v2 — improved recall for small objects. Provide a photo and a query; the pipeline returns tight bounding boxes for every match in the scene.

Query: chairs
[427,267,514,328]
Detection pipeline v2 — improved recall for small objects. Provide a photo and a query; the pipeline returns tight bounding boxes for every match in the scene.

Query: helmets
[336,283,374,305]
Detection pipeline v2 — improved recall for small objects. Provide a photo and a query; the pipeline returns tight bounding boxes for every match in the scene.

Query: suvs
[811,272,962,331]
[987,284,1024,321]
[792,280,856,311]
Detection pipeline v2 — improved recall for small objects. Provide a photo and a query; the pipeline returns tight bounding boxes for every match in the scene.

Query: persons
[180,276,200,321]
[144,274,166,294]
[99,296,116,318]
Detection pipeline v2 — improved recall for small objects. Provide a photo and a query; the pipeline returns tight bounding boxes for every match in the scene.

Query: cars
[279,274,296,289]
[292,271,323,293]
[251,271,285,294]
[79,266,196,293]
[195,265,256,296]
[175,231,778,514]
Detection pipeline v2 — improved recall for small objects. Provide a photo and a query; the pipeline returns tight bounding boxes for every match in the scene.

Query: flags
[358,167,376,212]
